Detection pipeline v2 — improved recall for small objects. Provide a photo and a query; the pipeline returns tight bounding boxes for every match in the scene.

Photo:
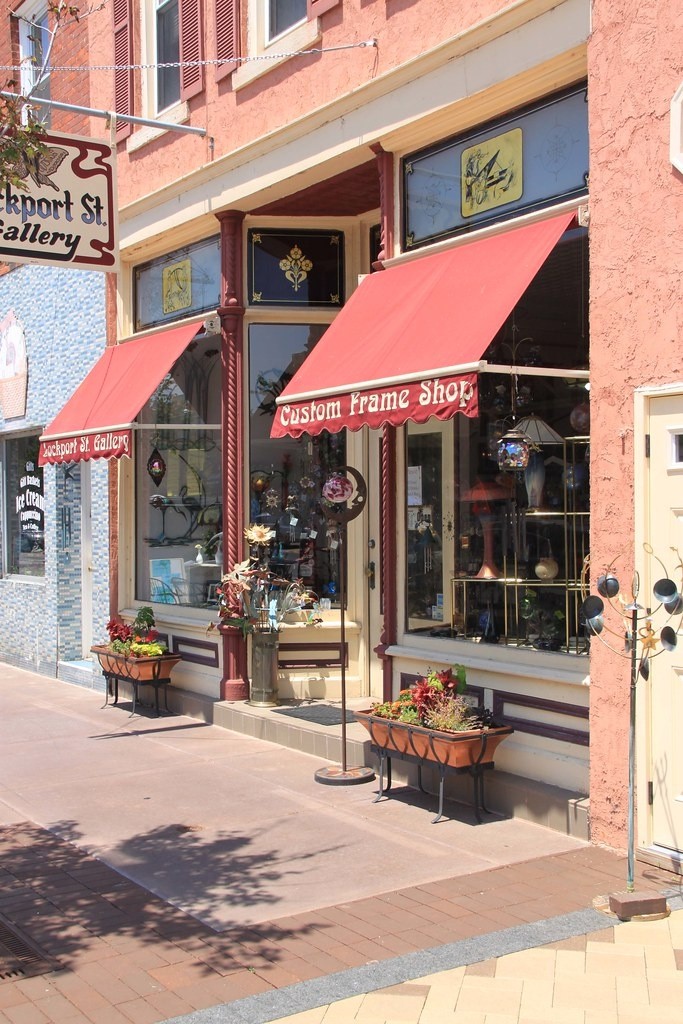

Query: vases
[352,709,513,768]
[90,644,182,682]
[250,627,281,708]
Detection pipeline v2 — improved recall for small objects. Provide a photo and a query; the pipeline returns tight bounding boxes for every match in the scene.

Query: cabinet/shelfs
[451,434,591,655]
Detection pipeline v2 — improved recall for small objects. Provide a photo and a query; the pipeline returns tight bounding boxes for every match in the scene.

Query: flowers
[205,521,324,632]
[368,663,496,731]
[105,605,169,659]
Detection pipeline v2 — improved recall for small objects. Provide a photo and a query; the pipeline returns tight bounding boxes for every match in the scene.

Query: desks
[194,561,296,582]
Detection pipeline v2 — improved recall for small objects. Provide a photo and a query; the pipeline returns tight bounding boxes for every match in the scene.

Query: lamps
[496,429,529,469]
[322,474,353,503]
[513,412,565,513]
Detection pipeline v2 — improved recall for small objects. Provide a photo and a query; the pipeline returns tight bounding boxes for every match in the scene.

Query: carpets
[271,704,357,726]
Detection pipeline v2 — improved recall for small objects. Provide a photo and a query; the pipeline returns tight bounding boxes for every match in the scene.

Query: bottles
[484,586,501,644]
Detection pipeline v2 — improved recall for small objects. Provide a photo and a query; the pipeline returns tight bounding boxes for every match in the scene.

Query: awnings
[269,206,588,439]
[36,309,219,465]
